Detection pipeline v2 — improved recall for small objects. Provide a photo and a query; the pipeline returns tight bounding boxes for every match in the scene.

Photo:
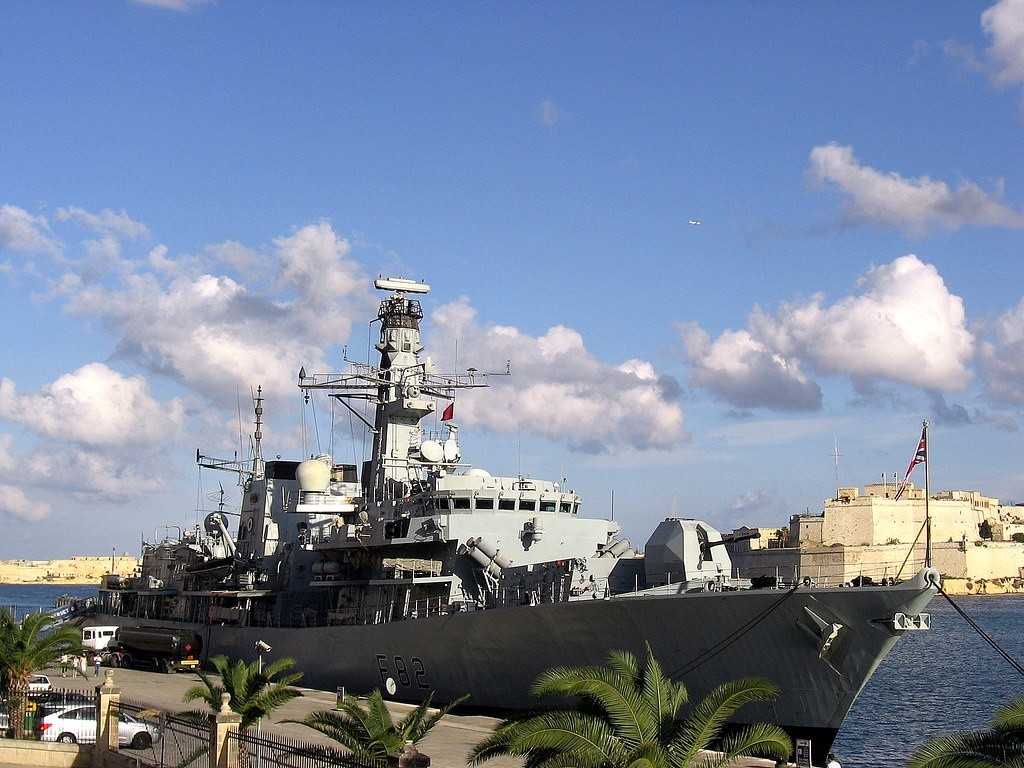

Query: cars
[0,673,161,750]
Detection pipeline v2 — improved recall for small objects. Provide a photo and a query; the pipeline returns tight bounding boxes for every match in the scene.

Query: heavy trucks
[101,626,203,673]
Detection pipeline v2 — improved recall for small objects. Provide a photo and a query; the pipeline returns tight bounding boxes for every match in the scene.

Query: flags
[440,401,456,421]
[893,420,927,501]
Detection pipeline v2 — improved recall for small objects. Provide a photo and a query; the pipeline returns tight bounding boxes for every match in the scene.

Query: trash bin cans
[22,702,37,730]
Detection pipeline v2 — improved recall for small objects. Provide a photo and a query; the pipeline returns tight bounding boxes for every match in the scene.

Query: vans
[81,626,121,652]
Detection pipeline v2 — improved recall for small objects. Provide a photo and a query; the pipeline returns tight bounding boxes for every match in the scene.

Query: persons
[93,653,102,678]
[71,655,79,679]
[80,652,88,677]
[60,652,68,678]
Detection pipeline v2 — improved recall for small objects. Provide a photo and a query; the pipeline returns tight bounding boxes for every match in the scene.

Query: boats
[61,259,943,766]
